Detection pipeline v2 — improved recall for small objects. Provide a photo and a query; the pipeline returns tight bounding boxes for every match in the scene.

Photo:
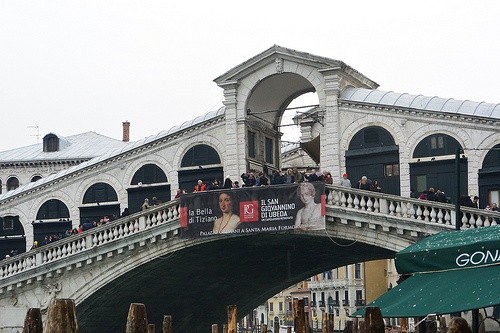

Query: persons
[174,167,383,213]
[27,196,162,255]
[295,182,322,227]
[446,317,472,333]
[212,190,242,233]
[6,248,23,268]
[412,187,500,228]
